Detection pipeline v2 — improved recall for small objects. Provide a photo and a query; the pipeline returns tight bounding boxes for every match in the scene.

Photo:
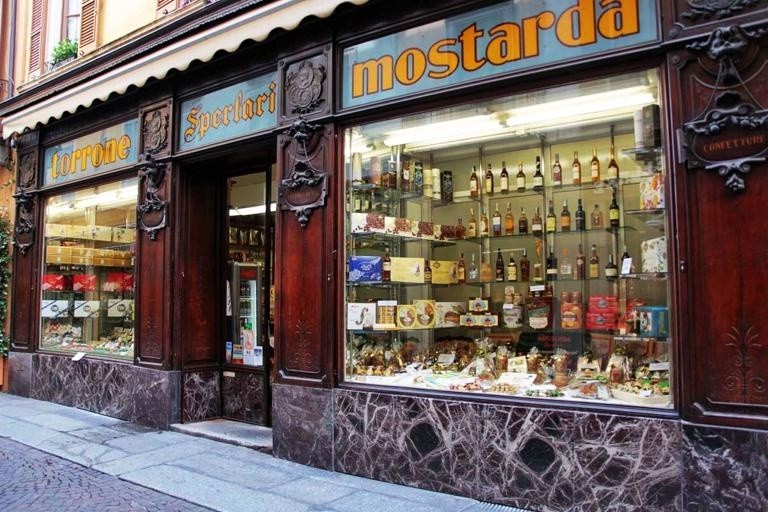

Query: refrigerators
[224,261,262,347]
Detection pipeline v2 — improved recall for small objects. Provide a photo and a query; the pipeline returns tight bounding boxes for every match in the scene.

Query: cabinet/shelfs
[480,133,552,334]
[612,113,669,341]
[346,145,485,379]
[541,121,626,340]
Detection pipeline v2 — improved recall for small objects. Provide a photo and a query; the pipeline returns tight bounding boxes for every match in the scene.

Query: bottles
[346,180,382,213]
[382,144,631,284]
[240,282,251,329]
